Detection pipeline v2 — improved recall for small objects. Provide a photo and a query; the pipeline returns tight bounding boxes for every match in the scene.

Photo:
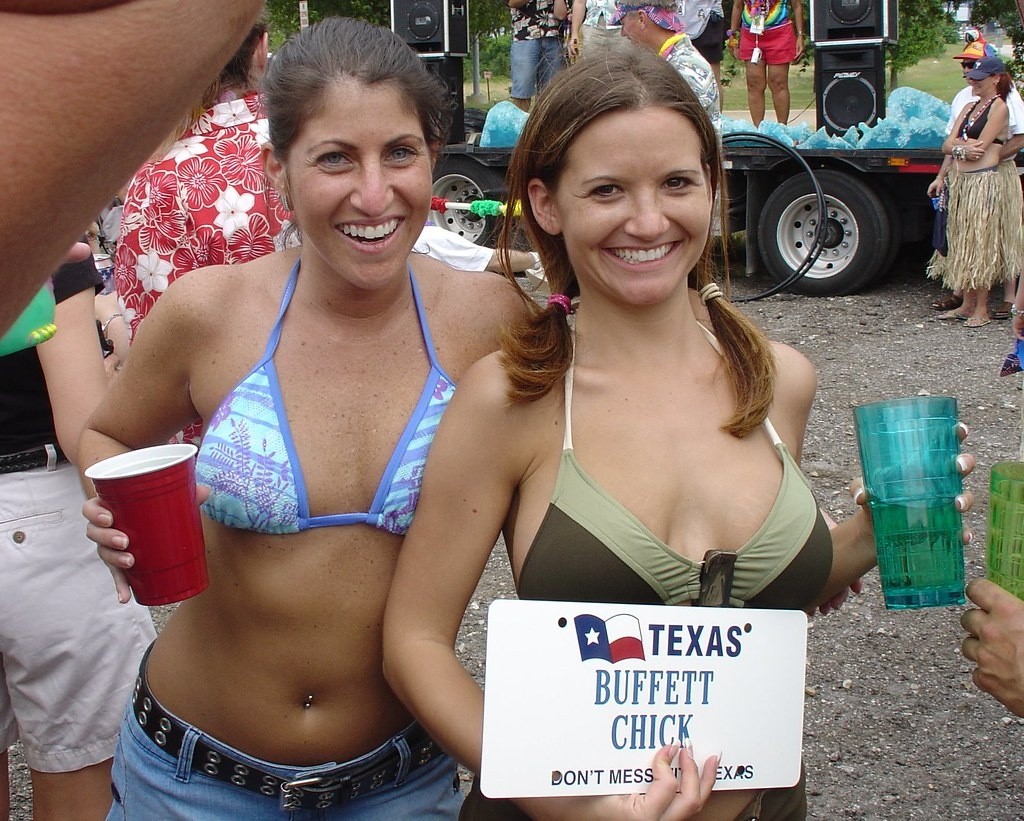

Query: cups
[853,397,966,610]
[988,462,1024,603]
[85,443,210,605]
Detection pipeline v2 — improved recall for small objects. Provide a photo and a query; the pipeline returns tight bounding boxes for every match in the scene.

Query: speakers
[390,0,469,57]
[420,57,466,144]
[814,43,886,140]
[809,0,899,46]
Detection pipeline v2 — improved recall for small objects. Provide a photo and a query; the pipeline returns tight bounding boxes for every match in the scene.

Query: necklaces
[962,92,1001,141]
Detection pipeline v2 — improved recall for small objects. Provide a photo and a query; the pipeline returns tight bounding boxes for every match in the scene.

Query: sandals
[990,302,1014,319]
[930,295,963,310]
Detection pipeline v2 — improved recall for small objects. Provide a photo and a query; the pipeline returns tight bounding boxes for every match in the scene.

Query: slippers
[937,311,969,320]
[963,317,991,327]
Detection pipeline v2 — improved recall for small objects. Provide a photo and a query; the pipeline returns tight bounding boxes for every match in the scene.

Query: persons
[931,40,1024,319]
[960,576,1024,716]
[726,0,805,148]
[966,21,984,40]
[504,0,724,113]
[82,20,860,821]
[927,56,1014,325]
[1,0,545,354]
[609,0,725,235]
[0,230,161,821]
[1010,260,1024,341]
[383,43,978,821]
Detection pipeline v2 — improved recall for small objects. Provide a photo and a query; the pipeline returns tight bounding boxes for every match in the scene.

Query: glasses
[961,61,977,69]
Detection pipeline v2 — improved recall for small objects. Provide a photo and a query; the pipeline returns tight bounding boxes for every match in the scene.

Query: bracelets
[100,313,124,332]
[796,28,805,36]
[727,30,736,36]
[1010,304,1024,319]
[725,39,738,48]
[936,176,944,178]
[951,146,966,161]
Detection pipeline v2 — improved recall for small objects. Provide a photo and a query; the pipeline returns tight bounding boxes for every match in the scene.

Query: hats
[953,42,995,60]
[962,56,1007,80]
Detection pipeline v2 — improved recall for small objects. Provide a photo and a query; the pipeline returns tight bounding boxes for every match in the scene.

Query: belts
[132,639,444,811]
[0,445,69,474]
